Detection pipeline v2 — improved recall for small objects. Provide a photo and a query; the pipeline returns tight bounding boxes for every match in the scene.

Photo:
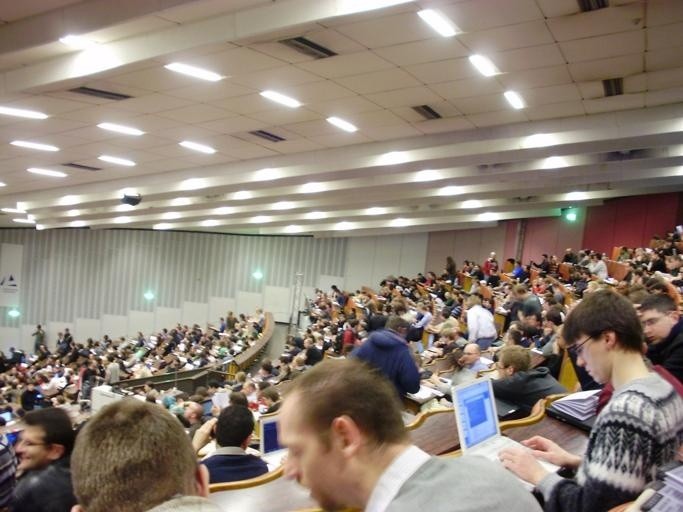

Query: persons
[197,404,268,484]
[71,397,223,512]
[499,290,683,511]
[0,307,263,458]
[0,408,76,510]
[264,228,683,414]
[278,355,541,512]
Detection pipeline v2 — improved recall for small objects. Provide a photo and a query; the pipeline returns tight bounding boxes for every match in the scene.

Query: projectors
[122,196,141,206]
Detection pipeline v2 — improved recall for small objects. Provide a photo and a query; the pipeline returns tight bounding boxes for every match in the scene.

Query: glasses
[570,336,591,355]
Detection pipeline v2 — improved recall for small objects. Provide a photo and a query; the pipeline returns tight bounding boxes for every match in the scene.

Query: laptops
[451,376,563,492]
[261,418,289,466]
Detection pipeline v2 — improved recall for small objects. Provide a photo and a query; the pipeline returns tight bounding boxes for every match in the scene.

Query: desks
[192,238,683,512]
